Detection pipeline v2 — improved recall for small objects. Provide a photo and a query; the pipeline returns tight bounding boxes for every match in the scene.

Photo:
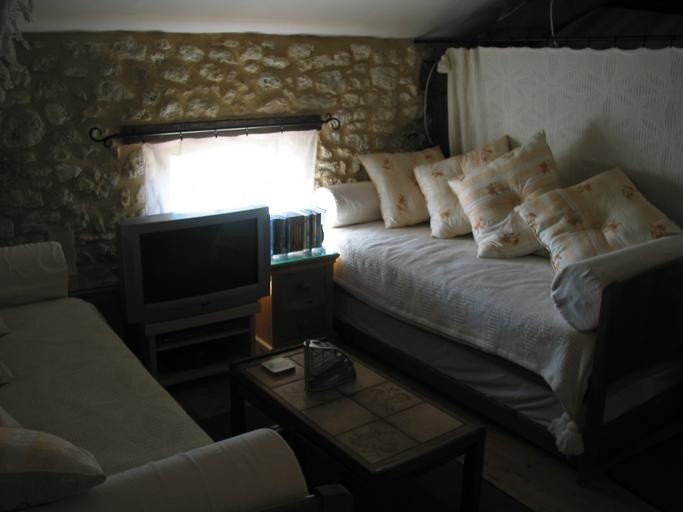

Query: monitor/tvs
[115,204,271,324]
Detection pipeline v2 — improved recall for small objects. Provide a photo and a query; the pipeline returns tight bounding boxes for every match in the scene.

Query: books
[270,208,322,255]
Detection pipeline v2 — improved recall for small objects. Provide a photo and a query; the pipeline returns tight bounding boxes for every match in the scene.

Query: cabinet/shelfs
[253,252,341,352]
[144,302,260,385]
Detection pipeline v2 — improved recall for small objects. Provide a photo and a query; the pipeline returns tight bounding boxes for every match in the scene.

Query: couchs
[1,241,310,512]
[315,178,682,474]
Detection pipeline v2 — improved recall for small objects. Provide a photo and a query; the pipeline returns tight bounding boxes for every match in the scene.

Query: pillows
[514,166,682,270]
[0,426,105,512]
[413,135,512,238]
[447,130,563,258]
[358,146,446,226]
